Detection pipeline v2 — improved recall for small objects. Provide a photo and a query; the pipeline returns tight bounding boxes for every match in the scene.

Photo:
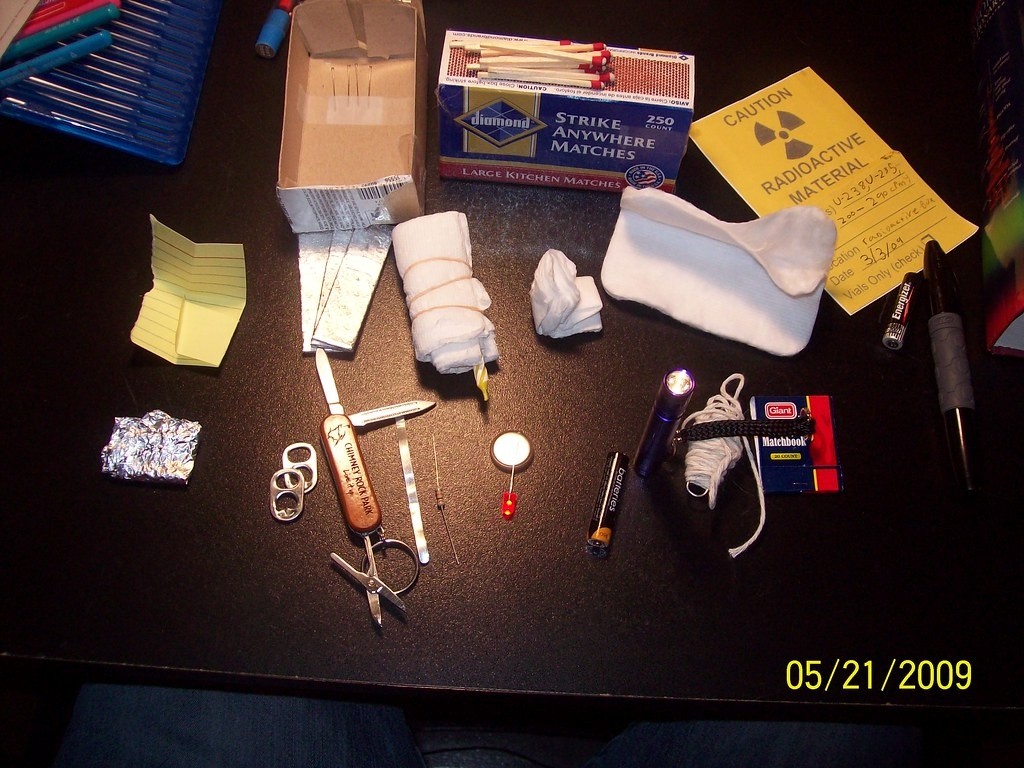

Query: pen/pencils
[0,30,112,88]
[0,3,119,64]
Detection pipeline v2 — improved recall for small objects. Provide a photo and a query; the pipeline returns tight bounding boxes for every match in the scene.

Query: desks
[0,0,1023,712]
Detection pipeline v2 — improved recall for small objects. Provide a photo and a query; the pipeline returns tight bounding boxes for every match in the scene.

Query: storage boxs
[276,0,429,235]
[435,30,696,196]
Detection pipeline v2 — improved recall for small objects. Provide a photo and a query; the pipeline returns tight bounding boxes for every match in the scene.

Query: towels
[391,210,499,374]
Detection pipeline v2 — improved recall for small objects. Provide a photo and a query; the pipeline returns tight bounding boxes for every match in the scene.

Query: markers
[925,240,986,493]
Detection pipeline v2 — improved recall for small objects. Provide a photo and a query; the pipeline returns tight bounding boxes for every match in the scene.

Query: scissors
[331,536,407,628]
